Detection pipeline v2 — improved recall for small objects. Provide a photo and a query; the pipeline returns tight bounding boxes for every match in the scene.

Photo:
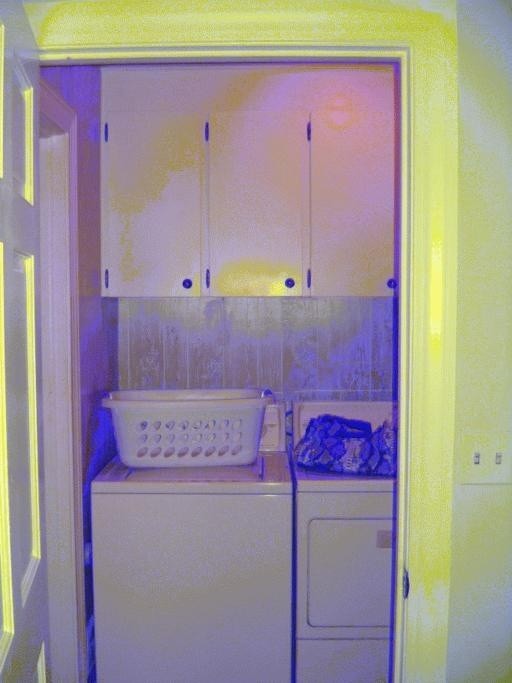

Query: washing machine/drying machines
[289,397,395,682]
[87,397,296,683]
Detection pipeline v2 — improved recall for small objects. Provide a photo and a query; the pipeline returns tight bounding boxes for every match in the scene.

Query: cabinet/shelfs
[208,107,304,298]
[309,108,396,298]
[100,110,204,299]
